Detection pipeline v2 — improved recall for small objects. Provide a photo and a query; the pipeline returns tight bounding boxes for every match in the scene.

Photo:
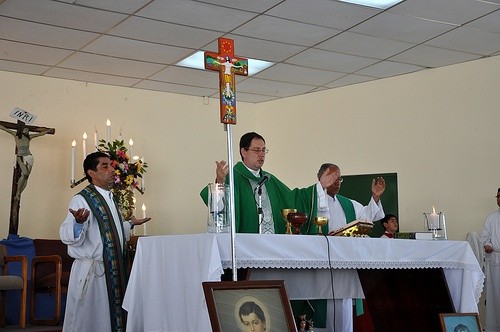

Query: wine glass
[282,208,298,234]
[314,216,326,236]
[287,212,308,234]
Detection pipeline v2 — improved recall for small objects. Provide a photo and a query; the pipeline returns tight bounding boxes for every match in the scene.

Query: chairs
[0,238,76,328]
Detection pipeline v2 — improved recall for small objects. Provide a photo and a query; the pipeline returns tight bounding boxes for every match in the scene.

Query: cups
[207,183,231,233]
[424,212,440,232]
[430,212,447,240]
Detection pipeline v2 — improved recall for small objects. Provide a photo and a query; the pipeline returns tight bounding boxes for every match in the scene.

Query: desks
[120,231,487,332]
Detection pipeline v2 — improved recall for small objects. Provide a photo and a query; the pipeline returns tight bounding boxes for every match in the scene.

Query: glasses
[248,147,269,155]
[336,177,344,183]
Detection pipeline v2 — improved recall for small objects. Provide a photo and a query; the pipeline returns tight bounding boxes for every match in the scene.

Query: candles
[429,206,439,228]
[212,183,221,210]
[141,203,147,235]
[70,118,145,190]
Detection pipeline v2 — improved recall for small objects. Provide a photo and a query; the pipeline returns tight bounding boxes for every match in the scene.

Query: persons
[214,55,243,99]
[200,132,340,235]
[238,302,266,332]
[481,188,500,332]
[290,163,398,332]
[0,124,54,200]
[60,152,151,332]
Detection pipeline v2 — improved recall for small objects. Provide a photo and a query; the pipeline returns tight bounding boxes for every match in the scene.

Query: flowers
[96,139,148,219]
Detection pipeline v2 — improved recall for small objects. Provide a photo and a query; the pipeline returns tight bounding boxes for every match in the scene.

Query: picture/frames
[201,279,298,332]
[438,312,484,332]
